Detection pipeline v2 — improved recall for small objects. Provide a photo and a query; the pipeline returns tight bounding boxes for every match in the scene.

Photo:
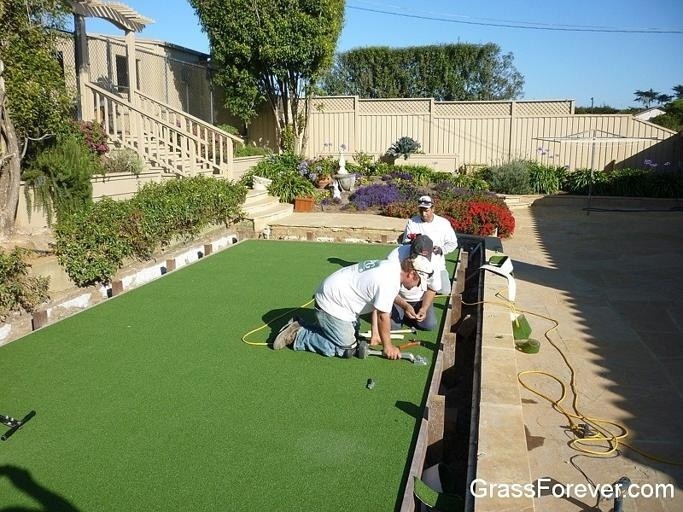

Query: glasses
[416,270,434,278]
[418,200,431,204]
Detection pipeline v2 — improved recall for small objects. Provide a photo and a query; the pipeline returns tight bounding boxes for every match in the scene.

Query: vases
[294,195,314,211]
[314,174,332,189]
[333,174,355,191]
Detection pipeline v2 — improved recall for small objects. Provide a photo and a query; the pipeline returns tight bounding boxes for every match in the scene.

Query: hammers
[368,326,417,334]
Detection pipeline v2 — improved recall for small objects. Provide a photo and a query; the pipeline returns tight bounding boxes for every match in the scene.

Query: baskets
[294,199,314,211]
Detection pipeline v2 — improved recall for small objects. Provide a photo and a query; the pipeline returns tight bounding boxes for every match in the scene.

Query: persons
[385,234,442,331]
[272,252,435,358]
[402,194,459,295]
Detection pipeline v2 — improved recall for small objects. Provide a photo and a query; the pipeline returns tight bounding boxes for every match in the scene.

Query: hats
[411,234,433,291]
[418,196,433,208]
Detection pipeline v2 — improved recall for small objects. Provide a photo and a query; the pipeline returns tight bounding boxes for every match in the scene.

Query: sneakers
[273,315,302,349]
